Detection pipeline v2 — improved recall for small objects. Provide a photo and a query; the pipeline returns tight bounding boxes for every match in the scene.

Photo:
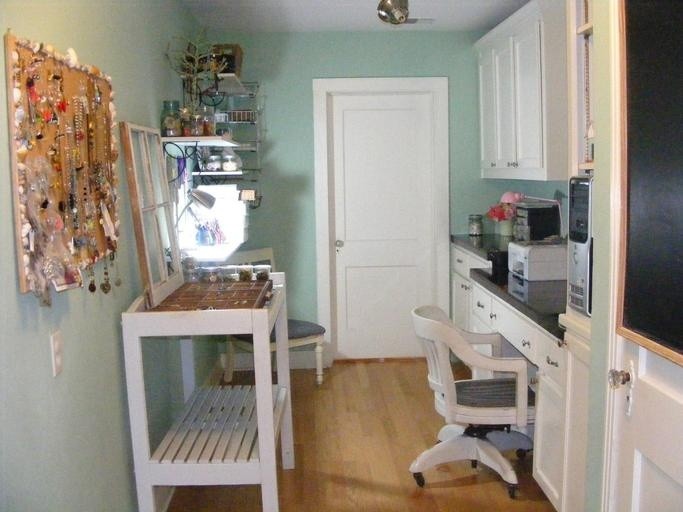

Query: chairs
[408,304,539,498]
[199,246,326,385]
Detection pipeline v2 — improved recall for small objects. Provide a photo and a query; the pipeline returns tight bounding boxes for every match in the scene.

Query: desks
[468,266,569,510]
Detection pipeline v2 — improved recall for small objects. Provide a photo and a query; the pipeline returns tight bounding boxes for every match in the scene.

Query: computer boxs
[566,175,591,315]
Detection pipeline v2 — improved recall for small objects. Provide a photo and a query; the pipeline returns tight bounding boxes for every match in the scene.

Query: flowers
[486,202,522,223]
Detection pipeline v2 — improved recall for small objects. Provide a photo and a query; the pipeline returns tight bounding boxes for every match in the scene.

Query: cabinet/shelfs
[449,232,524,371]
[477,1,569,181]
[163,70,265,209]
[121,266,296,512]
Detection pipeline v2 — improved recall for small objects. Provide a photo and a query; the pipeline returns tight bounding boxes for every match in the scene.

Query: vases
[494,216,514,236]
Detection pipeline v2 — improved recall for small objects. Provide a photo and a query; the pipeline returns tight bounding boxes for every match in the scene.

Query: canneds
[468,214,483,236]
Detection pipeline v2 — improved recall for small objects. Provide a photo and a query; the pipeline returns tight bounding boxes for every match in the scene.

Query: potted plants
[165,8,230,136]
